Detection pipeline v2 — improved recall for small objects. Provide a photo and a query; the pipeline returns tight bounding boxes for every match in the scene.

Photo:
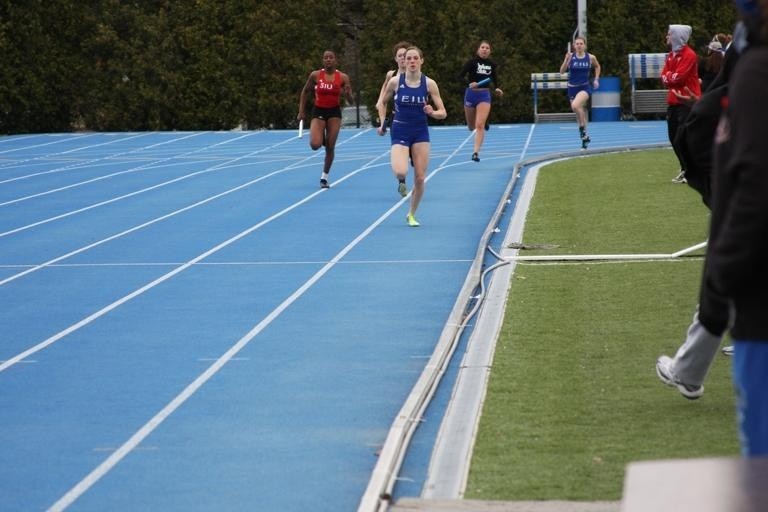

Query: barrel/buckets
[591,76,621,122]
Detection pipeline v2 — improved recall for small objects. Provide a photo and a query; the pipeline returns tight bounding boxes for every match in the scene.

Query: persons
[376,41,414,166]
[559,37,601,149]
[296,50,354,189]
[377,45,448,228]
[654,0,767,456]
[459,37,504,162]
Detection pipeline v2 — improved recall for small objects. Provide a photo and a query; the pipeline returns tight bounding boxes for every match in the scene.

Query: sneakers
[656,356,704,400]
[579,127,590,149]
[320,179,329,187]
[472,152,480,161]
[398,183,406,197]
[406,216,419,227]
